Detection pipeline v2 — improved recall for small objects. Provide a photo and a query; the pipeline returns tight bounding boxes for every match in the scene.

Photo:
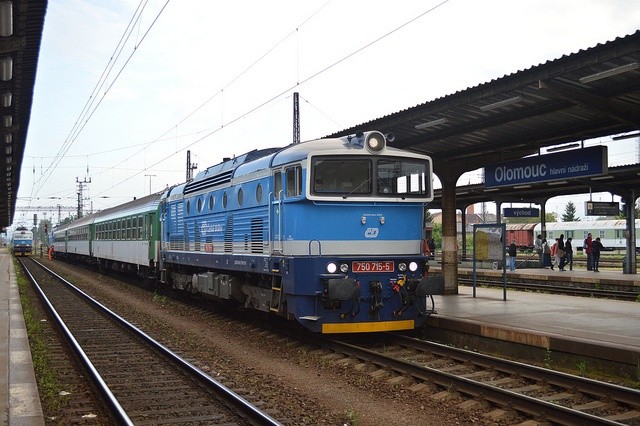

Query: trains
[10,227,33,257]
[48,129,447,339]
[457,219,640,255]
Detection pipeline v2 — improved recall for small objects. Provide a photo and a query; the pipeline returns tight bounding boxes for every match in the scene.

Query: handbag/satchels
[557,249,564,258]
[545,243,550,254]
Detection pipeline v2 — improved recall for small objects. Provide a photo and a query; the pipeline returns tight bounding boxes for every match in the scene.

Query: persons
[564,237,573,270]
[314,178,323,192]
[585,232,593,271]
[535,234,544,262]
[509,239,516,271]
[558,234,565,271]
[551,237,561,270]
[540,238,552,269]
[591,237,604,272]
[48,245,54,261]
[422,237,435,263]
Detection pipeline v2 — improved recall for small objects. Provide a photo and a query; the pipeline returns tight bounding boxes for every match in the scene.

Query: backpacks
[583,239,588,249]
